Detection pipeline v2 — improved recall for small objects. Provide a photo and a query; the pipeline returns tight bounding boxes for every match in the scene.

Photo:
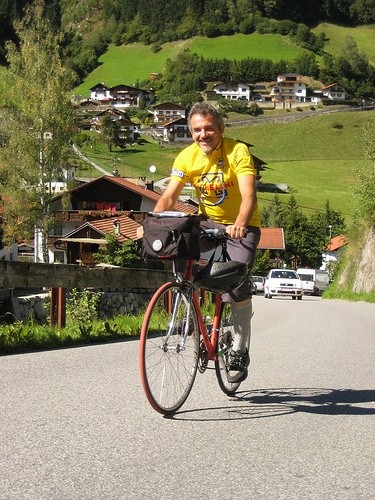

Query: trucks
[296,268,330,296]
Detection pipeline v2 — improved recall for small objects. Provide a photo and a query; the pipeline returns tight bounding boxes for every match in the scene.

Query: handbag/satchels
[141,217,201,261]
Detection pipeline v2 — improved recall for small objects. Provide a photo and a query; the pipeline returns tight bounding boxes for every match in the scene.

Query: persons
[134,102,261,382]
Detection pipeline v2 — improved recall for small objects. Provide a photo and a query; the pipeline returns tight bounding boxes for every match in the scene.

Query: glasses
[198,173,226,206]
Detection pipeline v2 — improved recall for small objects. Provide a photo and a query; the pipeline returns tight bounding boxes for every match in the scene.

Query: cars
[250,275,265,295]
[263,268,304,300]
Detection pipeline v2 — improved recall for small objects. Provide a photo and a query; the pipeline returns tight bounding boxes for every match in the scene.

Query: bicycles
[137,211,262,414]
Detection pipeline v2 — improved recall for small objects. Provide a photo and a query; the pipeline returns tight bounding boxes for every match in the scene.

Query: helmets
[193,260,250,294]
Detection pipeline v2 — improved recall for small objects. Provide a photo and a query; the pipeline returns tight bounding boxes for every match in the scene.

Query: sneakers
[227,348,250,383]
[178,316,202,336]
